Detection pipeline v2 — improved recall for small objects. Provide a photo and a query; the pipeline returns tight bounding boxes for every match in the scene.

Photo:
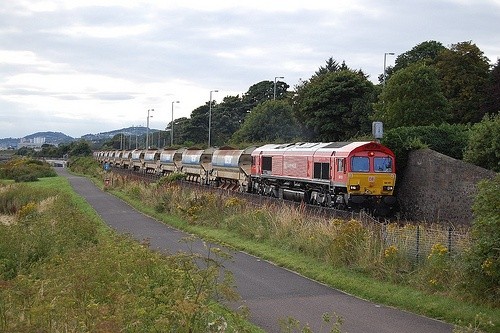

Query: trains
[91,138,395,212]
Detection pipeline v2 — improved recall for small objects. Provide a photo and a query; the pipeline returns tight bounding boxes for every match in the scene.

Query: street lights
[208,90,218,147]
[121,108,165,151]
[274,77,284,102]
[171,101,179,146]
[382,52,394,115]
